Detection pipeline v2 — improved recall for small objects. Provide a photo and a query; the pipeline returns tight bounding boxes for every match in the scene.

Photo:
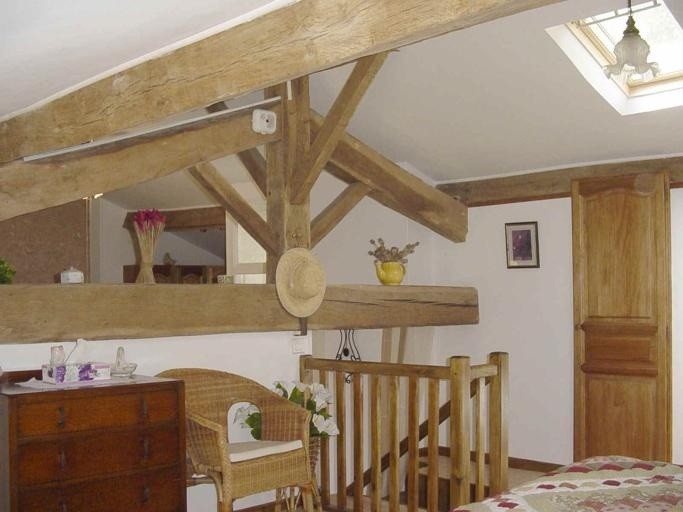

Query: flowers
[123,207,166,227]
[234,381,340,439]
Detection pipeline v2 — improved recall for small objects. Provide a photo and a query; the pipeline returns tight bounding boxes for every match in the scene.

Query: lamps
[601,0,661,80]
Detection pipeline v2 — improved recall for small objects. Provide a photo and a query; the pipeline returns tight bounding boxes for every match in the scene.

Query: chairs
[156,366,313,512]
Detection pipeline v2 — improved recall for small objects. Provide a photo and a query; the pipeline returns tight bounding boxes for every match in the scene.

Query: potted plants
[367,237,420,285]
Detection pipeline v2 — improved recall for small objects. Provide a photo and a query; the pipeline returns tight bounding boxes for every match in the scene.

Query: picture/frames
[503,221,541,270]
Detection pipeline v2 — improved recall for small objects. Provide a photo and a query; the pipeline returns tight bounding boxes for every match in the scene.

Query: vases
[130,222,164,285]
[308,440,320,474]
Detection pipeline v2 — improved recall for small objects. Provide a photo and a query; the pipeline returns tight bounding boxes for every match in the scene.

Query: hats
[275,246,327,318]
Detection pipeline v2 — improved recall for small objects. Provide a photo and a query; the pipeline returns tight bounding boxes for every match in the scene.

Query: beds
[446,455,683,512]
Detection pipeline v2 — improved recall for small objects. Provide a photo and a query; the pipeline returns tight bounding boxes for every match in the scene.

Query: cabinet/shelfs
[1,371,187,512]
